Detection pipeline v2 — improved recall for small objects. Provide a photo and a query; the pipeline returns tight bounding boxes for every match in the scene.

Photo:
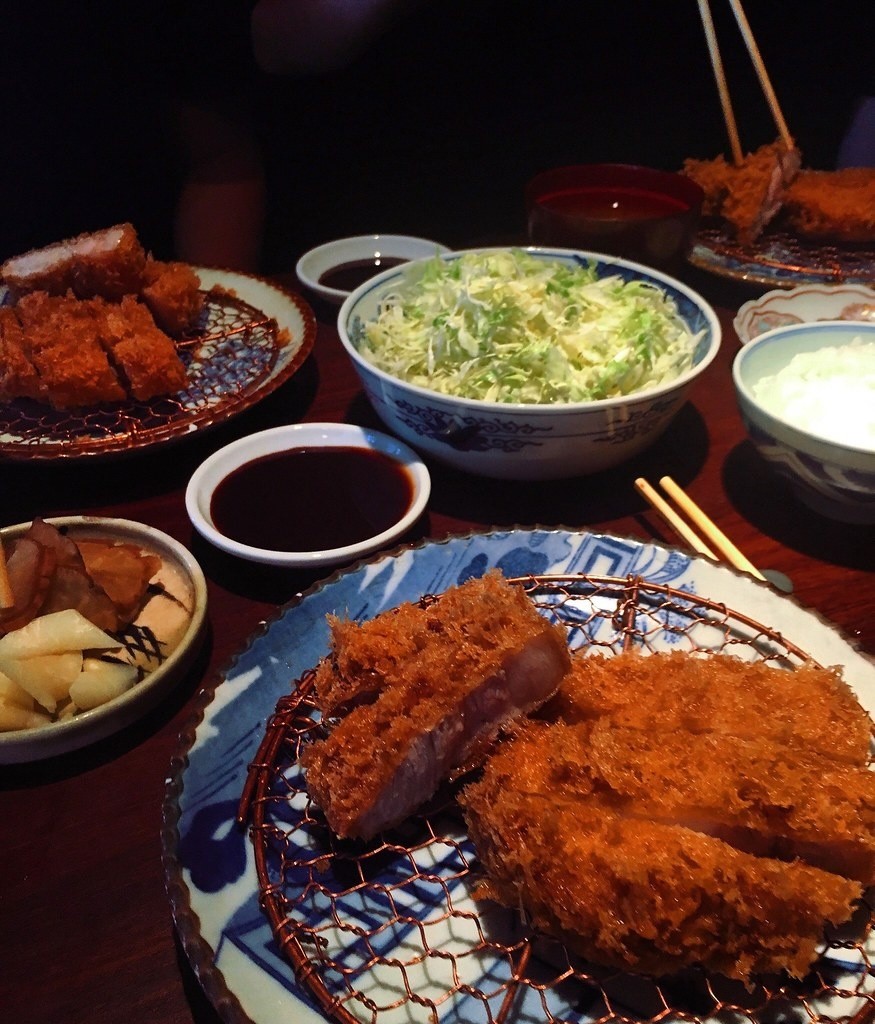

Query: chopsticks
[633,476,770,586]
[699,0,794,167]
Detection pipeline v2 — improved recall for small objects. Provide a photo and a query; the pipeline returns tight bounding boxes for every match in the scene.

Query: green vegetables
[351,244,704,405]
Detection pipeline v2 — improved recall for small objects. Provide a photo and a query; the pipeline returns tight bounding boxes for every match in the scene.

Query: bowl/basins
[0,515,209,765]
[524,162,704,285]
[337,246,723,482]
[733,320,875,529]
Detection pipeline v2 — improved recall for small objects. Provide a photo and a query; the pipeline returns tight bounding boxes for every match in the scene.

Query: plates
[732,286,875,345]
[185,423,433,568]
[295,235,453,303]
[683,224,875,289]
[0,265,317,464]
[159,523,875,1024]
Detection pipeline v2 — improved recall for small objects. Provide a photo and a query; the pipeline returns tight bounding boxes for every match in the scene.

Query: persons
[0,0,875,275]
